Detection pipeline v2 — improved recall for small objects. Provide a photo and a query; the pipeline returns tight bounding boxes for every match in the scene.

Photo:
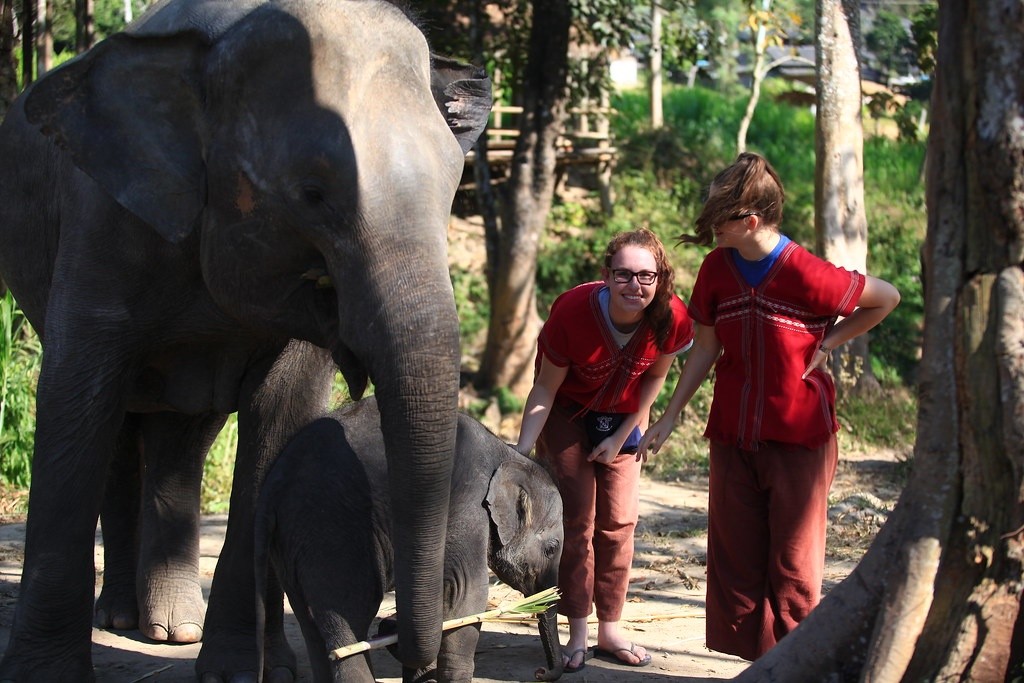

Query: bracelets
[819,343,832,354]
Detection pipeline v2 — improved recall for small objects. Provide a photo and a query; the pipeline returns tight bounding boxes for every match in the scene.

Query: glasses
[711,213,762,229]
[606,266,660,285]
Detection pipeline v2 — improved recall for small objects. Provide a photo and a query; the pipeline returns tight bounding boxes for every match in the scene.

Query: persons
[634,150,901,664]
[502,226,694,674]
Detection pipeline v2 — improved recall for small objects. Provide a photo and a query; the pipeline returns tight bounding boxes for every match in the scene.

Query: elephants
[0,0,493,682]
[252,388,565,683]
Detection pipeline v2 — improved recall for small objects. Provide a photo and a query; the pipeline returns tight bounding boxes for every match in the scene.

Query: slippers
[559,646,596,672]
[593,642,651,667]
